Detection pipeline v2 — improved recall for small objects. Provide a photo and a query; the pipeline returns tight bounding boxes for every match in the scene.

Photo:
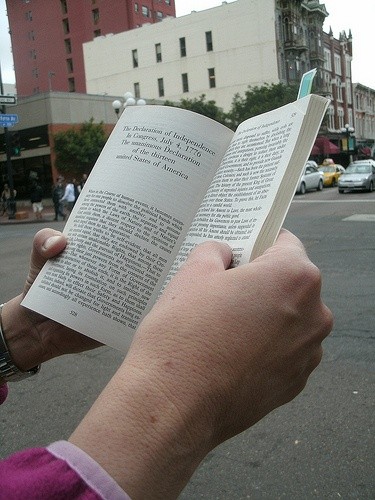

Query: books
[21,95,331,353]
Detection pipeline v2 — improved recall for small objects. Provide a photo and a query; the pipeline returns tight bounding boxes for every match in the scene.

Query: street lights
[341,124,355,164]
[112,91,147,120]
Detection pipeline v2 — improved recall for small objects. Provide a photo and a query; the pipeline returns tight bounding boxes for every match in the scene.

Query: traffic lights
[8,145,21,157]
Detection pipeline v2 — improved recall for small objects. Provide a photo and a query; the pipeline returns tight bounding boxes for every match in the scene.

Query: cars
[319,158,345,187]
[337,159,375,194]
[296,161,324,194]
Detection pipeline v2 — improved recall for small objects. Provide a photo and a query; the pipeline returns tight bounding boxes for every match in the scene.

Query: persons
[0,184,17,220]
[0,227,333,500]
[51,174,87,221]
[30,172,43,222]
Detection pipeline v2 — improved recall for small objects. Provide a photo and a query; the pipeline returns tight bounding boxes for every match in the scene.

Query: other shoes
[8,216,15,219]
[63,216,66,220]
[54,218,57,221]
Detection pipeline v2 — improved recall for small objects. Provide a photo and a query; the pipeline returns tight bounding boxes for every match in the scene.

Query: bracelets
[1,303,42,382]
